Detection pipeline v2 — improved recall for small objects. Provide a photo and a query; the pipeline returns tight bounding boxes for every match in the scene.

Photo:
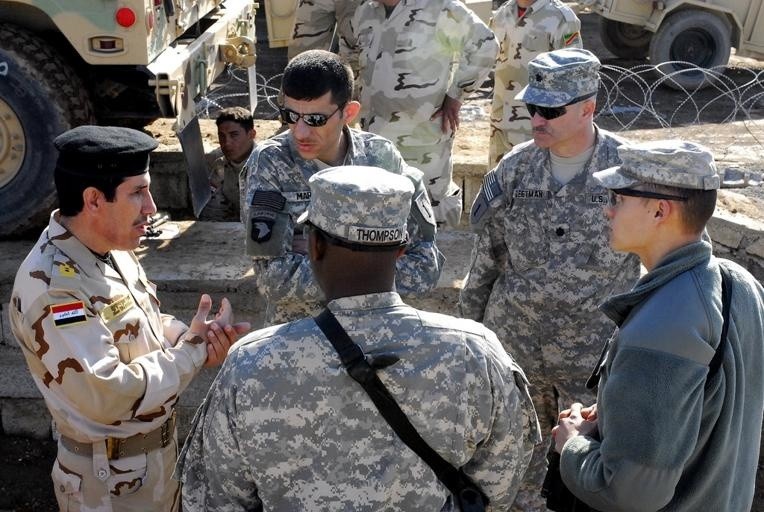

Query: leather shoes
[58,408,177,460]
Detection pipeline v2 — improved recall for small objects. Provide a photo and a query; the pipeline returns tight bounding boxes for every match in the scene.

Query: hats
[50,124,162,177]
[296,163,415,247]
[513,47,602,107]
[591,139,725,192]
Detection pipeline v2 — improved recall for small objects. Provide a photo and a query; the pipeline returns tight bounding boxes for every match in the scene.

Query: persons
[201,107,259,222]
[9,126,250,512]
[458,46,711,512]
[349,0,500,233]
[277,0,363,80]
[169,164,542,511]
[236,49,446,327]
[552,138,763,512]
[488,0,583,173]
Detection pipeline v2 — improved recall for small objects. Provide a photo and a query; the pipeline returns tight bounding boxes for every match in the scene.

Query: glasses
[604,186,687,208]
[302,223,355,253]
[277,101,345,127]
[523,92,598,120]
